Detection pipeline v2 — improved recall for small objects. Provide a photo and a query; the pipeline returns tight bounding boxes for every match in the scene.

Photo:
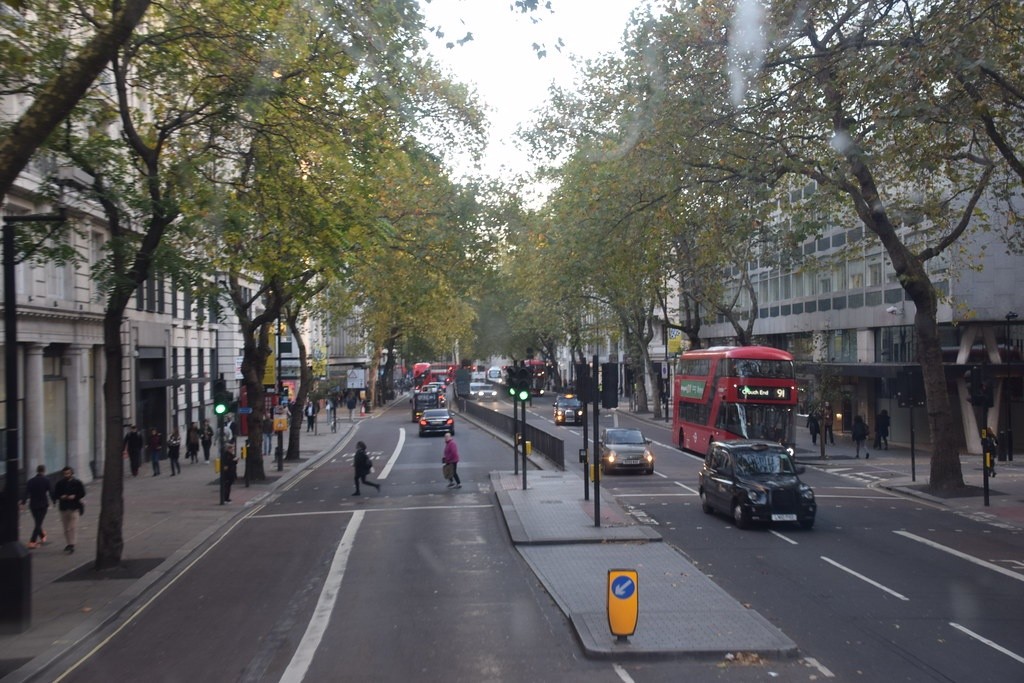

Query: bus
[672,346,797,455]
[487,367,502,383]
[410,363,455,422]
[523,360,545,396]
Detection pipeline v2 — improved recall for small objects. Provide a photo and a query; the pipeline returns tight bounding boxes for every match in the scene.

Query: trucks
[471,372,486,383]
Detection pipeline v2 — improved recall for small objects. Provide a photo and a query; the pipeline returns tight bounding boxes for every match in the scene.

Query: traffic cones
[360,404,367,416]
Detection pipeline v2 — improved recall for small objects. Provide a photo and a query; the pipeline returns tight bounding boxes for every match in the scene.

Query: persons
[806,410,820,445]
[121,414,241,502]
[20,465,57,548]
[981,427,999,478]
[51,466,86,552]
[852,415,870,459]
[264,377,414,456]
[821,401,836,446]
[874,410,890,450]
[444,433,462,489]
[351,441,381,496]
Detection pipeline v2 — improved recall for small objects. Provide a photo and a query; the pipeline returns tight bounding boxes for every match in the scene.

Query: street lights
[1006,311,1019,462]
[0,213,58,633]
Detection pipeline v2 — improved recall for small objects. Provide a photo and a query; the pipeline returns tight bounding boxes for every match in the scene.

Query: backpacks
[358,460,372,476]
[864,425,868,435]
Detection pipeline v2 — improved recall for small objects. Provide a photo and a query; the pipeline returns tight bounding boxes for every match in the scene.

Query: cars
[598,428,654,474]
[416,409,456,436]
[477,384,498,403]
[469,383,485,395]
[698,438,817,531]
[552,394,583,425]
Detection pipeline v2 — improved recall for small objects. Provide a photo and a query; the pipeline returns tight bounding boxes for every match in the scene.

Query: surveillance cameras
[887,306,897,313]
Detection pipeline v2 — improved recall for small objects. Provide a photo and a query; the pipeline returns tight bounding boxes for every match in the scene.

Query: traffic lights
[507,366,518,396]
[213,379,228,415]
[964,366,980,405]
[517,367,530,400]
[983,382,993,407]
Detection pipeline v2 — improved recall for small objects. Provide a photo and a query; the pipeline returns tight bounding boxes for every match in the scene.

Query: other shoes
[376,483,381,492]
[64,545,74,551]
[205,461,210,464]
[28,541,37,548]
[454,484,462,489]
[446,482,454,488]
[40,530,46,543]
[856,455,859,458]
[153,473,161,476]
[196,460,198,463]
[191,461,194,464]
[865,453,869,458]
[170,474,175,476]
[885,447,888,450]
[352,491,361,496]
[177,470,181,473]
[879,447,881,450]
[992,471,996,477]
[225,498,231,502]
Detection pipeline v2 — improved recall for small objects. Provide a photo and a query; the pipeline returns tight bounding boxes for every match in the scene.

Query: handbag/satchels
[443,463,454,478]
[873,432,878,447]
[77,500,84,515]
[185,451,191,458]
